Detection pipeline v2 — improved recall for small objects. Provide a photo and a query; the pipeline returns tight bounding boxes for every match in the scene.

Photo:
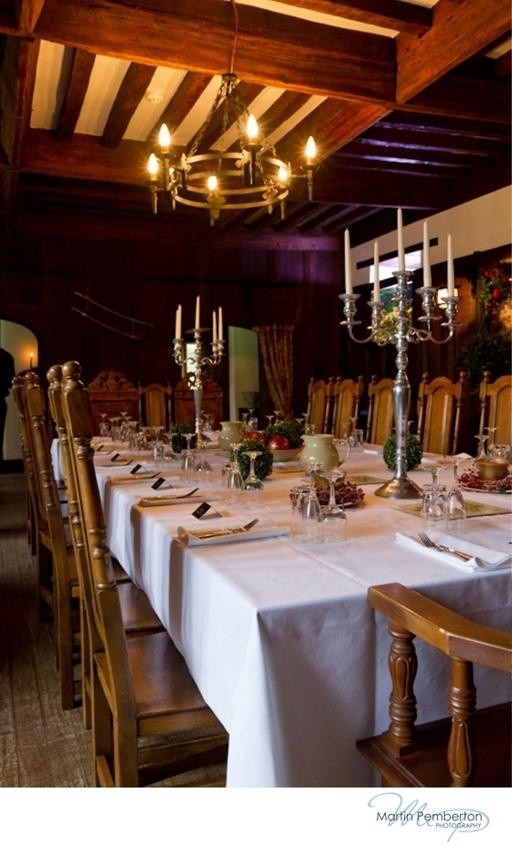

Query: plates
[460,483,512,494]
[321,501,353,509]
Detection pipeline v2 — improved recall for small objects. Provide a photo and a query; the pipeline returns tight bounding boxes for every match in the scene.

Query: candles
[342,208,460,296]
[169,296,226,342]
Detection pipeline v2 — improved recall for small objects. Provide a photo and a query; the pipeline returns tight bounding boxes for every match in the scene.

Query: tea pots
[297,433,350,475]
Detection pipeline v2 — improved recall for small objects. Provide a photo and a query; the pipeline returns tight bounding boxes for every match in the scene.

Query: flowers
[470,255,512,343]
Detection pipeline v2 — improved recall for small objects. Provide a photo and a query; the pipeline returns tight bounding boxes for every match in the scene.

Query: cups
[477,458,506,480]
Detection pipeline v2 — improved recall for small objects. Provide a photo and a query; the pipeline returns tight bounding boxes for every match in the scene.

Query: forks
[197,518,259,538]
[417,532,479,562]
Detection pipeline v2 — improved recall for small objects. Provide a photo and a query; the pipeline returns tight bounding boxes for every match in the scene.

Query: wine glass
[243,408,365,452]
[289,462,349,543]
[420,457,466,522]
[93,410,265,506]
[473,426,510,459]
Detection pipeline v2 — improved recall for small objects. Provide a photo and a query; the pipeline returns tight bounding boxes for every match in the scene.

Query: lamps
[144,0,321,227]
